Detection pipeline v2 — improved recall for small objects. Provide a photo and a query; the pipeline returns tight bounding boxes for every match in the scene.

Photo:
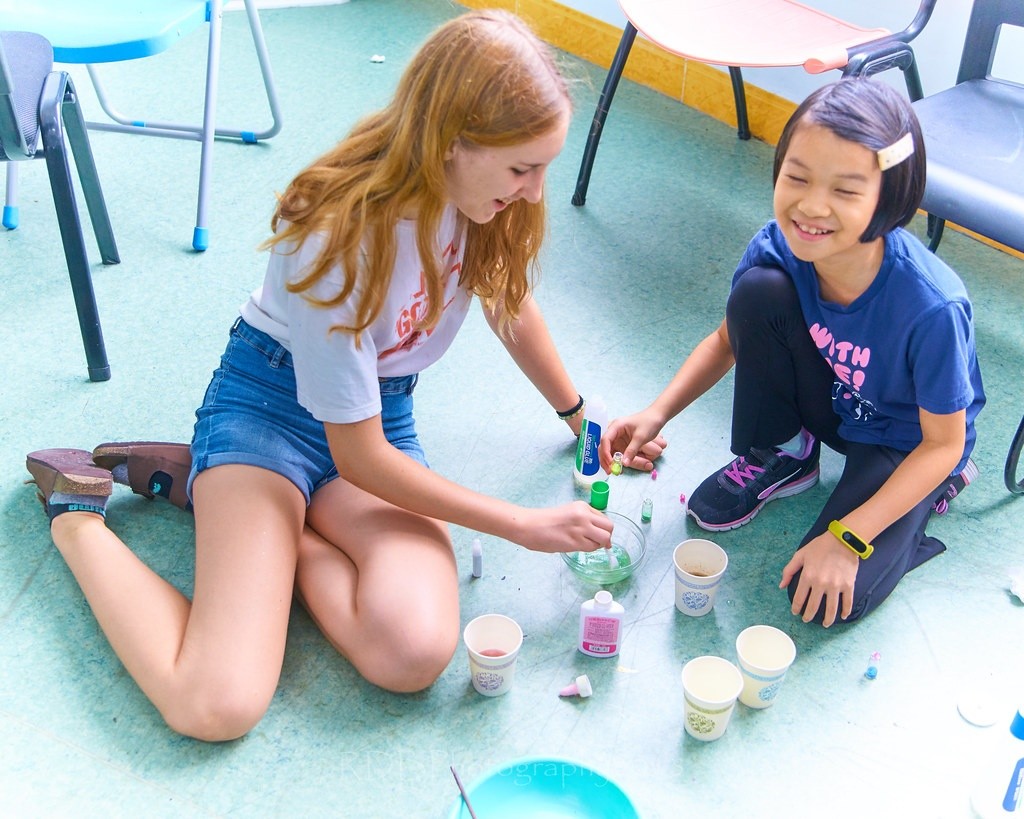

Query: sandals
[92,441,194,509]
[23,448,113,516]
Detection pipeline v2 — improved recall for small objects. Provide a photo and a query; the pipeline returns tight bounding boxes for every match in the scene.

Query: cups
[735,625,796,709]
[681,656,744,742]
[672,539,728,618]
[463,614,523,697]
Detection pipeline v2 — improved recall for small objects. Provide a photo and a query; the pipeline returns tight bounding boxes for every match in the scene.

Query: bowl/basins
[560,511,647,584]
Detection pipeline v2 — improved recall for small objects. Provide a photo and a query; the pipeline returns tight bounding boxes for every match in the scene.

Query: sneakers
[930,456,979,516]
[686,427,821,533]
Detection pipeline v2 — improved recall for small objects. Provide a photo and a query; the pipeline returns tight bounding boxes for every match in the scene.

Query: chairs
[570,1,936,240]
[0,28,121,382]
[840,0,1023,493]
[4,0,282,252]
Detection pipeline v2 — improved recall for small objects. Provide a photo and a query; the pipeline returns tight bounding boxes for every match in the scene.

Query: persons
[599,76,985,629]
[27,10,665,745]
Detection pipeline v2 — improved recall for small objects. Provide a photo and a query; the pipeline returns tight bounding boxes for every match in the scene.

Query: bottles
[577,590,625,658]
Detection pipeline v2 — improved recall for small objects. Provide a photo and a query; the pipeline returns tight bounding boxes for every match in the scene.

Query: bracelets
[557,395,587,421]
[828,520,874,560]
